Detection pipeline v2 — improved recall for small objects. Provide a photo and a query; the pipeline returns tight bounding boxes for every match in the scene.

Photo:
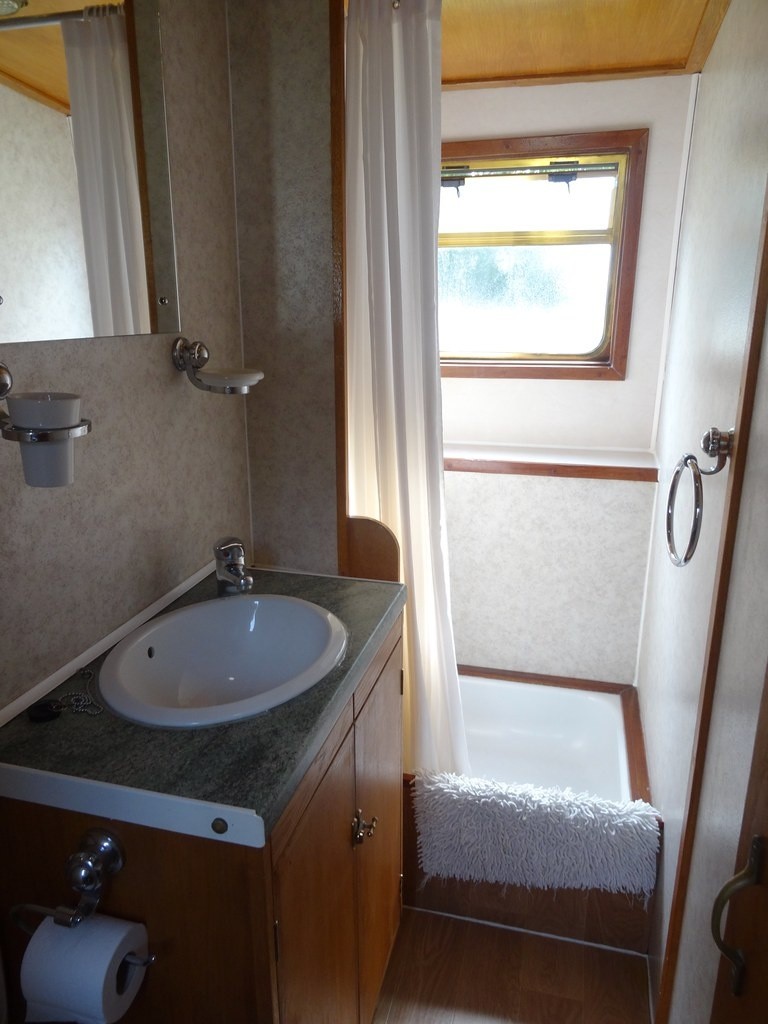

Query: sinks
[98,593,348,728]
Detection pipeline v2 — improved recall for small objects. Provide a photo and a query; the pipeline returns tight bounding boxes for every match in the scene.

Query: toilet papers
[20,912,149,1024]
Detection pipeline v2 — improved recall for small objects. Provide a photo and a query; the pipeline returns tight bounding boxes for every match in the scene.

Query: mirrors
[0,0,182,343]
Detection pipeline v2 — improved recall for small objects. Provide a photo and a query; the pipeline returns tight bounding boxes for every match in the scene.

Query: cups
[6,392,82,487]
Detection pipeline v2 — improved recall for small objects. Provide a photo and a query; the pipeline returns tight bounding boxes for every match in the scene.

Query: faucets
[212,536,254,594]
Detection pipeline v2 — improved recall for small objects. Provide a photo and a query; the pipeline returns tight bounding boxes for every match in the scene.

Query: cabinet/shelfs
[0,565,408,1024]
